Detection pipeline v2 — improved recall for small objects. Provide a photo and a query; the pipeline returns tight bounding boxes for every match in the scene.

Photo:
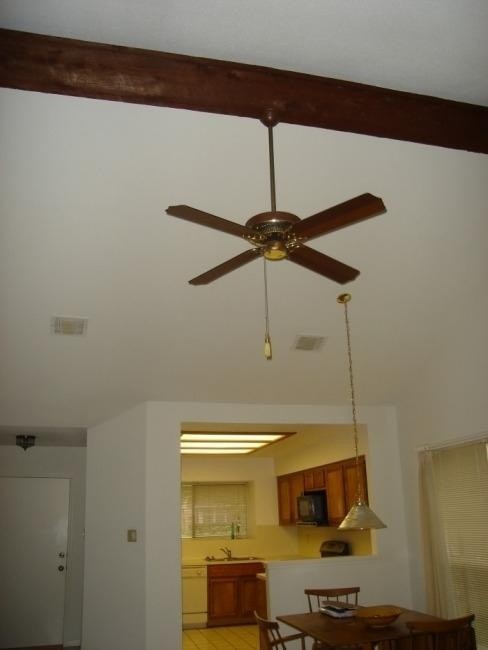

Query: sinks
[208,557,260,562]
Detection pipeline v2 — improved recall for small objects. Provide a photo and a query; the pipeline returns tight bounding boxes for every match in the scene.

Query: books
[318,598,364,619]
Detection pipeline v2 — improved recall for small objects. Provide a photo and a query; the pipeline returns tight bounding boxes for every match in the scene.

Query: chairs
[406,614,478,650]
[304,587,360,613]
[253,610,286,650]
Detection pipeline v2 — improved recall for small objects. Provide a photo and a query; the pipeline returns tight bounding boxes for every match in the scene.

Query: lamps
[261,240,289,360]
[337,293,389,531]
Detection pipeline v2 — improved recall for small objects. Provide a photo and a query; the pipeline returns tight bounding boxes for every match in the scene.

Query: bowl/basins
[352,605,404,629]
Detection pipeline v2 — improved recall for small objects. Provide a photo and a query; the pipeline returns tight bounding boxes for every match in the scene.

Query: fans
[164,113,386,285]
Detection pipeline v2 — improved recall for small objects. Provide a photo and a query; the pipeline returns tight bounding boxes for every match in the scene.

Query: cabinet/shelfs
[304,465,326,491]
[277,470,305,526]
[325,455,368,527]
[207,563,267,628]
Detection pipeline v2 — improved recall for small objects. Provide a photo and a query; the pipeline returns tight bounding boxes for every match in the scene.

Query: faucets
[219,546,231,557]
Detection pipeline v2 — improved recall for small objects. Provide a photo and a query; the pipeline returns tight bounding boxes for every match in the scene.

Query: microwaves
[296,494,327,522]
